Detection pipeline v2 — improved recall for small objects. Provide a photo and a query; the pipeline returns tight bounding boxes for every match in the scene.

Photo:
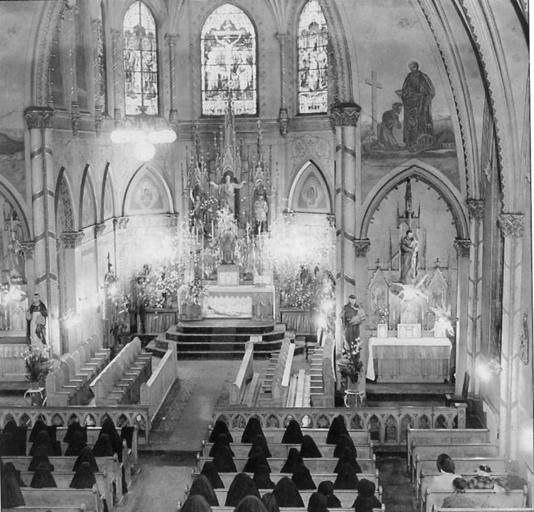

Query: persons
[419,452,499,509]
[377,102,402,146]
[190,187,205,239]
[177,416,383,512]
[214,31,240,82]
[0,417,135,511]
[399,229,418,280]
[208,175,247,217]
[395,61,436,146]
[25,292,50,357]
[252,193,270,234]
[342,295,365,349]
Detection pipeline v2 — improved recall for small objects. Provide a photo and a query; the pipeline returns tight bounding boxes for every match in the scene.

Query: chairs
[45,333,153,407]
[443,370,472,414]
[259,338,336,408]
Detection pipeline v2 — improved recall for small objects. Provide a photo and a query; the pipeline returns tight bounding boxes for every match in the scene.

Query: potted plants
[24,365,41,390]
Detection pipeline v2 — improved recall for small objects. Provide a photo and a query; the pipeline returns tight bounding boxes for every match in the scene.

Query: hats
[453,477,466,493]
[437,454,454,474]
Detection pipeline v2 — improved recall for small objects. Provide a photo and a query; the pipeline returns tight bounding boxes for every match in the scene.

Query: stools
[23,387,45,407]
